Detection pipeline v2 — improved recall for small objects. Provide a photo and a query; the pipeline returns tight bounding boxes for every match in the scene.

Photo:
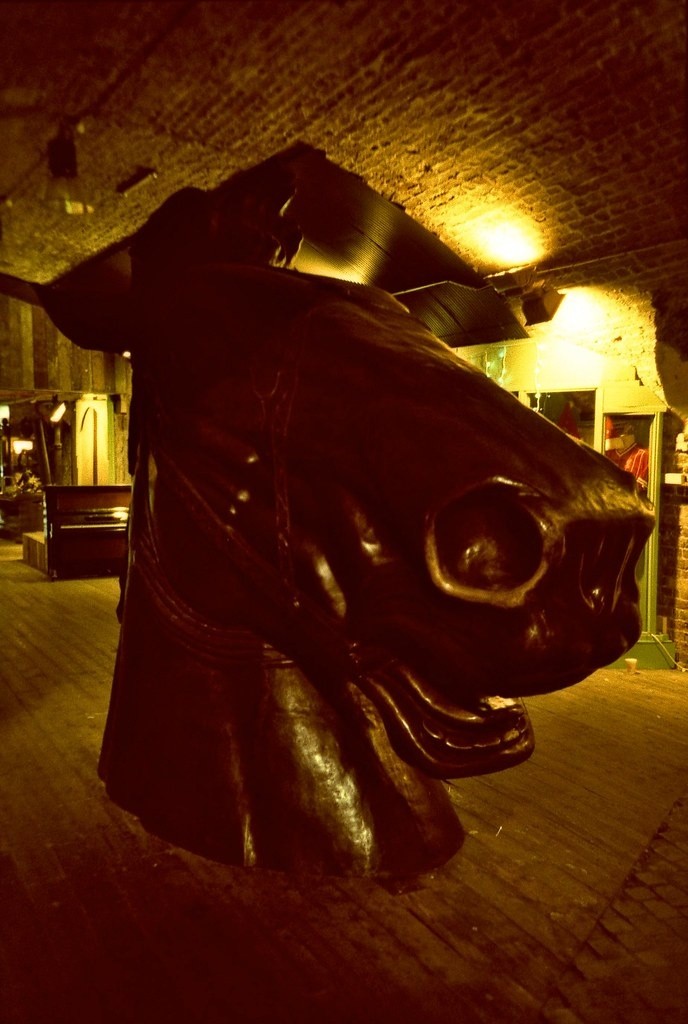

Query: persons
[605,433,648,492]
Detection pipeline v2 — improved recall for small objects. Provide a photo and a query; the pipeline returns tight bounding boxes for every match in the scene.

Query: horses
[31,255,658,882]
